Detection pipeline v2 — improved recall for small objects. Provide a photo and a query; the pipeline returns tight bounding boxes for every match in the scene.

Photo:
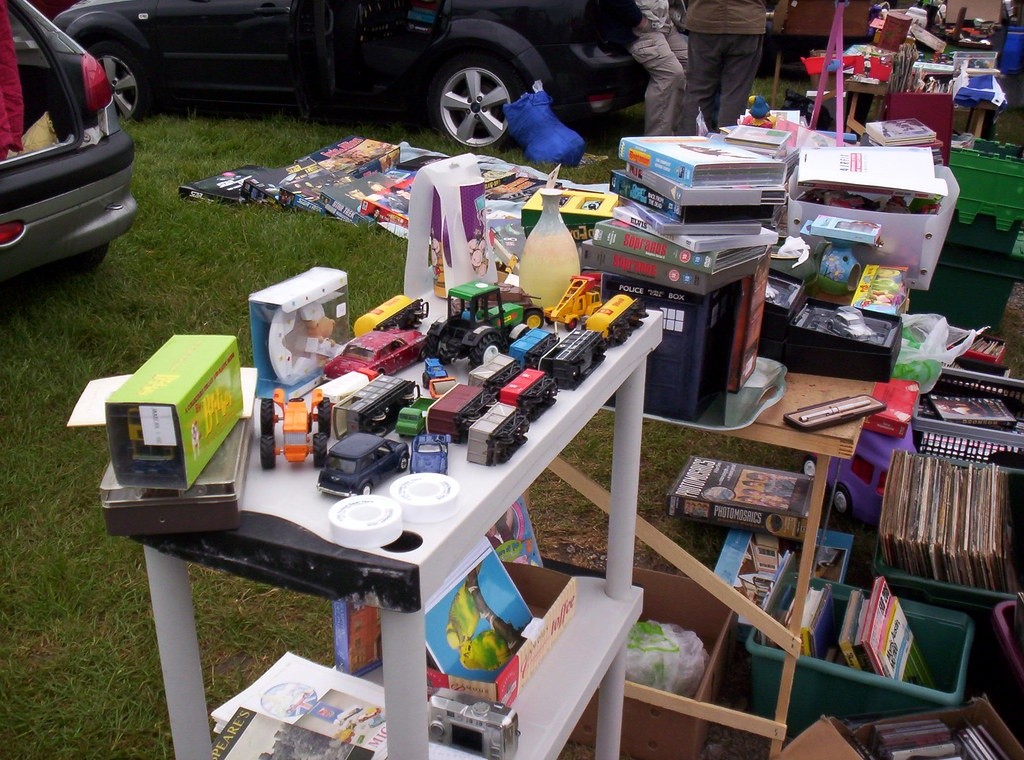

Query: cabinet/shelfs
[134,290,664,760]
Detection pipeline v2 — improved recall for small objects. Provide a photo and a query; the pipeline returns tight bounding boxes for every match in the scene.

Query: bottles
[518,188,581,308]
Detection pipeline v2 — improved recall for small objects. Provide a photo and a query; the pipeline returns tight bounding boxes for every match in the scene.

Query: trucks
[394,398,436,439]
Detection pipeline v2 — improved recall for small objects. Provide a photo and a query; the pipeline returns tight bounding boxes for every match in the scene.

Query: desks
[841,83,1002,142]
[523,372,885,760]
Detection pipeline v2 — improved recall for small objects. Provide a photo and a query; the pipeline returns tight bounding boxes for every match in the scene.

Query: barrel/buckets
[999,26,1024,75]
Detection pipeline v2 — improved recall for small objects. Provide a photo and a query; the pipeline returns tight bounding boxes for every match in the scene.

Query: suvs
[259,388,331,469]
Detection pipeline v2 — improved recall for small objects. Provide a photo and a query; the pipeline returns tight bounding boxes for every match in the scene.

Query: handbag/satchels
[624,618,712,696]
[888,314,977,394]
[502,91,586,168]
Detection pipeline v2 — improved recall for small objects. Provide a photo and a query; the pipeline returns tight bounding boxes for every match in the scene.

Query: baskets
[913,367,1024,469]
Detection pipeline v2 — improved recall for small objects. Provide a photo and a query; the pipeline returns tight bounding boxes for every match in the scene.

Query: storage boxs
[103,13,1023,760]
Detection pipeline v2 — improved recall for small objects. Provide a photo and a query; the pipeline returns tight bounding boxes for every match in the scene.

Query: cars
[317,431,409,497]
[410,433,448,475]
[324,328,426,379]
[51,0,650,152]
[0,0,138,286]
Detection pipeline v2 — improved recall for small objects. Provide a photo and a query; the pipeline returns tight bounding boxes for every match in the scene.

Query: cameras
[427,687,521,760]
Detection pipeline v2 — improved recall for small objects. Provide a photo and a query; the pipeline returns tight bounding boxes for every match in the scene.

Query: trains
[354,295,429,336]
[466,294,650,467]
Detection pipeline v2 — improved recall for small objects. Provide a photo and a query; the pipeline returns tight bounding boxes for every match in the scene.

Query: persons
[682,0,778,137]
[597,0,701,140]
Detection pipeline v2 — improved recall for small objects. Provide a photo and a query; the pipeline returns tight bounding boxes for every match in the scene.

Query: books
[761,397,1024,760]
[210,651,391,760]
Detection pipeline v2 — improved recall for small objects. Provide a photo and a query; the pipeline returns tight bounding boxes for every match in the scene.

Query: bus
[127,408,180,474]
[333,373,421,440]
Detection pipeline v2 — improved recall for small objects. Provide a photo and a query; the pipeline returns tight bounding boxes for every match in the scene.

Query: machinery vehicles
[450,282,545,330]
[422,358,457,399]
[543,274,603,333]
[425,280,531,369]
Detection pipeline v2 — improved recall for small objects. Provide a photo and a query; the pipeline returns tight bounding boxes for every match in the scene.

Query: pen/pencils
[798,400,871,422]
